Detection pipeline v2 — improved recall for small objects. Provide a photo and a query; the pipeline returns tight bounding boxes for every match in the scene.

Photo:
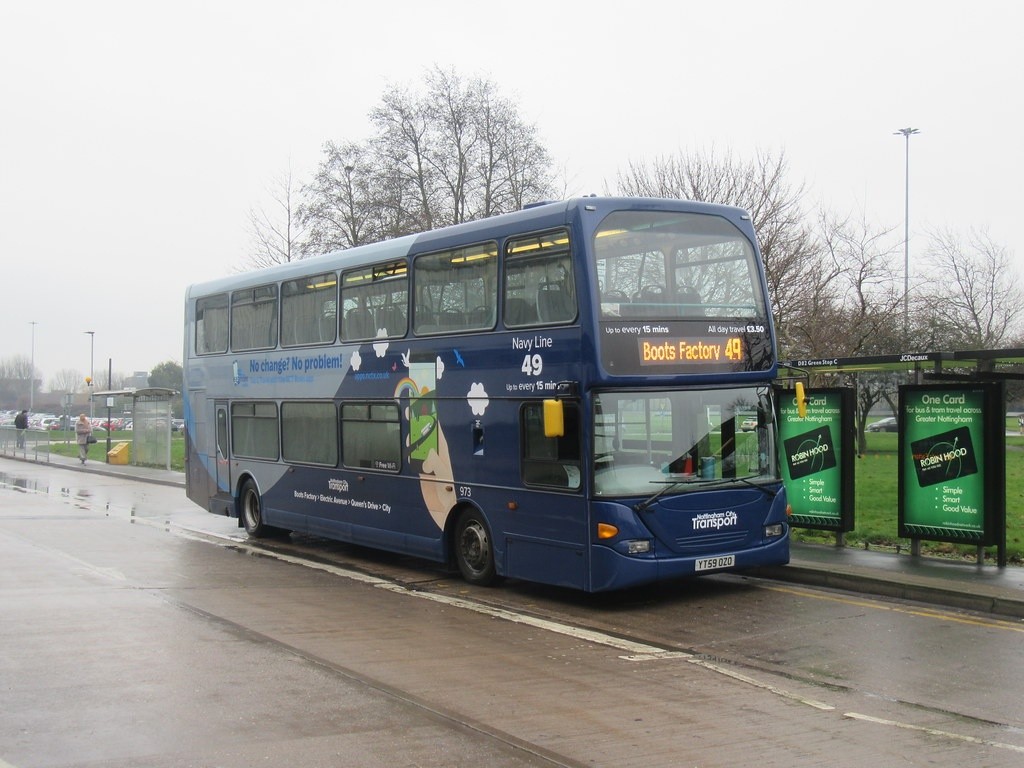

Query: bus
[181,198,812,592]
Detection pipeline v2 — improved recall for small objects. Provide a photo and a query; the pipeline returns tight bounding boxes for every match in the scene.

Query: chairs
[292,286,703,343]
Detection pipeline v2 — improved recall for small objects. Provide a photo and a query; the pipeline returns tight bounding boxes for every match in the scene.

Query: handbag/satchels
[86,432,97,443]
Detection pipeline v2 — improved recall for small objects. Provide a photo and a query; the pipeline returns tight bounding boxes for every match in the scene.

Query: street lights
[893,128,921,355]
[86,330,96,430]
[27,320,41,411]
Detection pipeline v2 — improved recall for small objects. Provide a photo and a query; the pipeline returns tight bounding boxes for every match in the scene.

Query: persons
[14,409,29,448]
[75,414,93,466]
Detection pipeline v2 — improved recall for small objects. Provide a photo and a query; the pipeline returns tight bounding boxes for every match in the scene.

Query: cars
[867,416,899,434]
[0,409,185,432]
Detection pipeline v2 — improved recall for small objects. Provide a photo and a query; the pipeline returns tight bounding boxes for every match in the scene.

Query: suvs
[742,416,758,432]
